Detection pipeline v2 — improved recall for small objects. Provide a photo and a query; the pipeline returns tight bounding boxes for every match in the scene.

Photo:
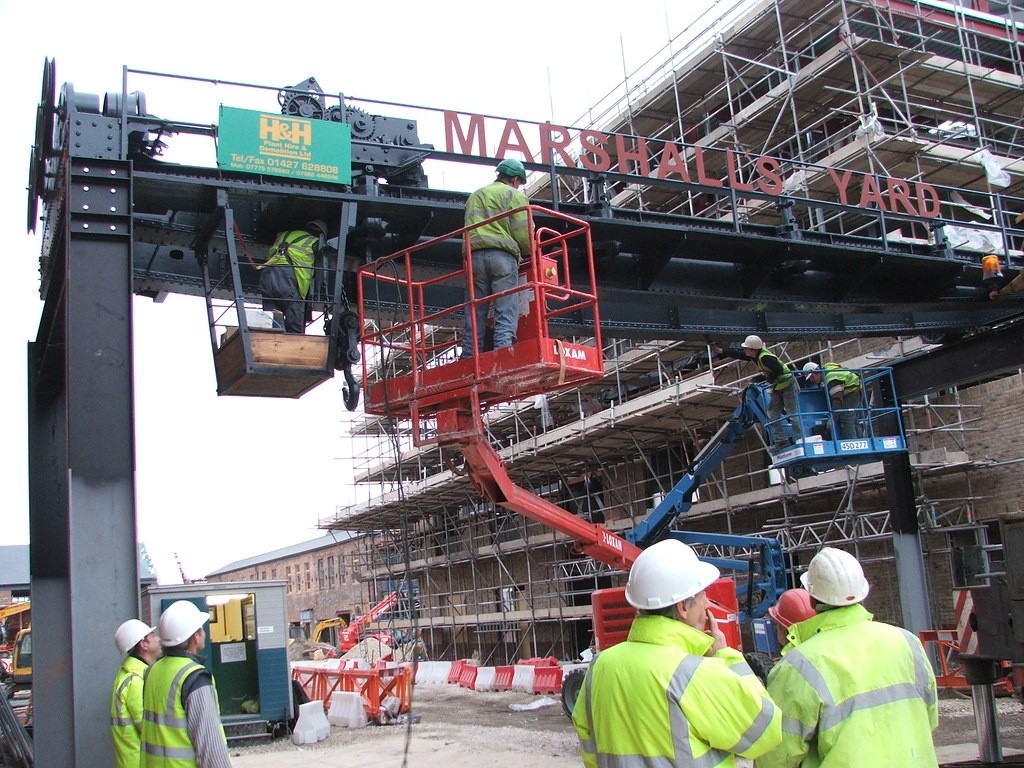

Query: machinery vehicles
[357,204,907,718]
[0,600,32,733]
[290,617,346,658]
[328,589,421,662]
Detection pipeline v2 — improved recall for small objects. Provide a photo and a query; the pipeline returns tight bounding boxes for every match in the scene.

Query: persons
[459,159,534,360]
[803,362,863,440]
[140,600,233,768]
[258,215,338,334]
[711,335,804,451]
[768,588,821,647]
[111,619,162,768]
[571,538,785,768]
[753,547,939,768]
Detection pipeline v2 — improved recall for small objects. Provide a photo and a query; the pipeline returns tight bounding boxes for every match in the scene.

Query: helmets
[803,362,819,380]
[768,587,820,630]
[306,218,328,238]
[800,546,869,606]
[494,159,526,184]
[741,335,763,350]
[115,618,157,655]
[624,537,722,611]
[159,600,210,647]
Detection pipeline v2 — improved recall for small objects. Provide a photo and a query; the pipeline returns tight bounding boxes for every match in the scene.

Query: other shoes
[768,439,790,452]
